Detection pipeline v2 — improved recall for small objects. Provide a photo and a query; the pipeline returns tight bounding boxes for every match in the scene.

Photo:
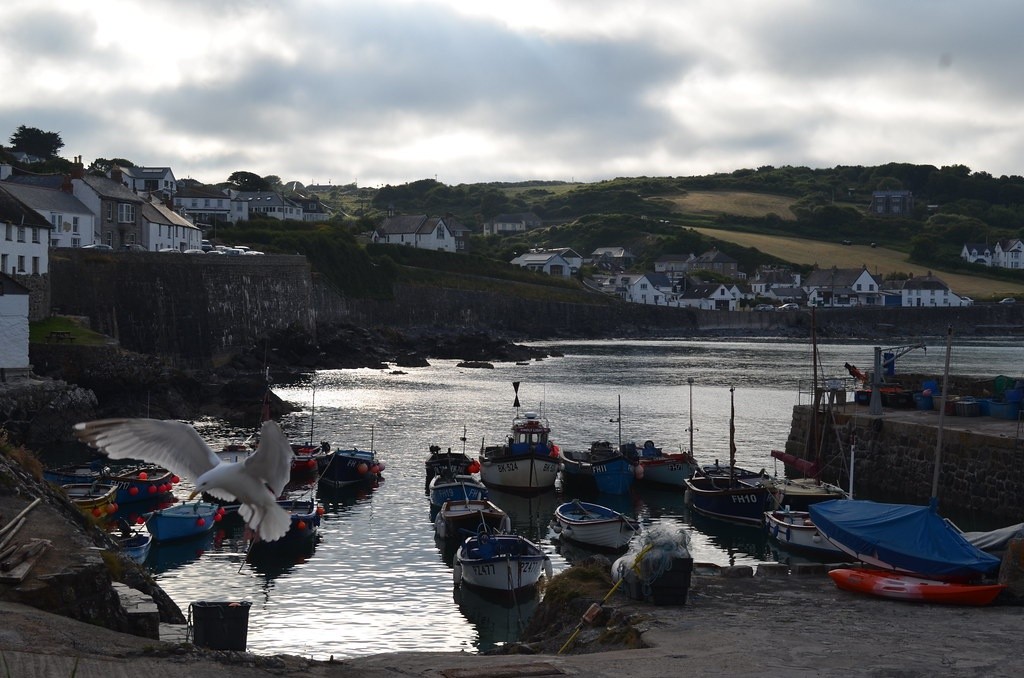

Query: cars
[184,240,264,256]
[81,243,113,250]
[961,296,974,305]
[117,243,148,252]
[998,297,1017,304]
[778,302,799,311]
[754,304,778,312]
[158,247,182,253]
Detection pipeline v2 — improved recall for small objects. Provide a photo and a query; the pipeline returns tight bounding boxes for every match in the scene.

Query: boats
[425,298,1009,610]
[41,378,388,569]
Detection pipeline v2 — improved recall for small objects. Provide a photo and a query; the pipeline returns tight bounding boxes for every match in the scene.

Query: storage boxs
[855,390,1020,419]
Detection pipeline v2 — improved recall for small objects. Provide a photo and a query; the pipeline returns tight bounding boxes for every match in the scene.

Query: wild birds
[72,417,297,544]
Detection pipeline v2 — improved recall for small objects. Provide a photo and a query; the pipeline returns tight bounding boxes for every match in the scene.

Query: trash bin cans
[191,601,253,652]
